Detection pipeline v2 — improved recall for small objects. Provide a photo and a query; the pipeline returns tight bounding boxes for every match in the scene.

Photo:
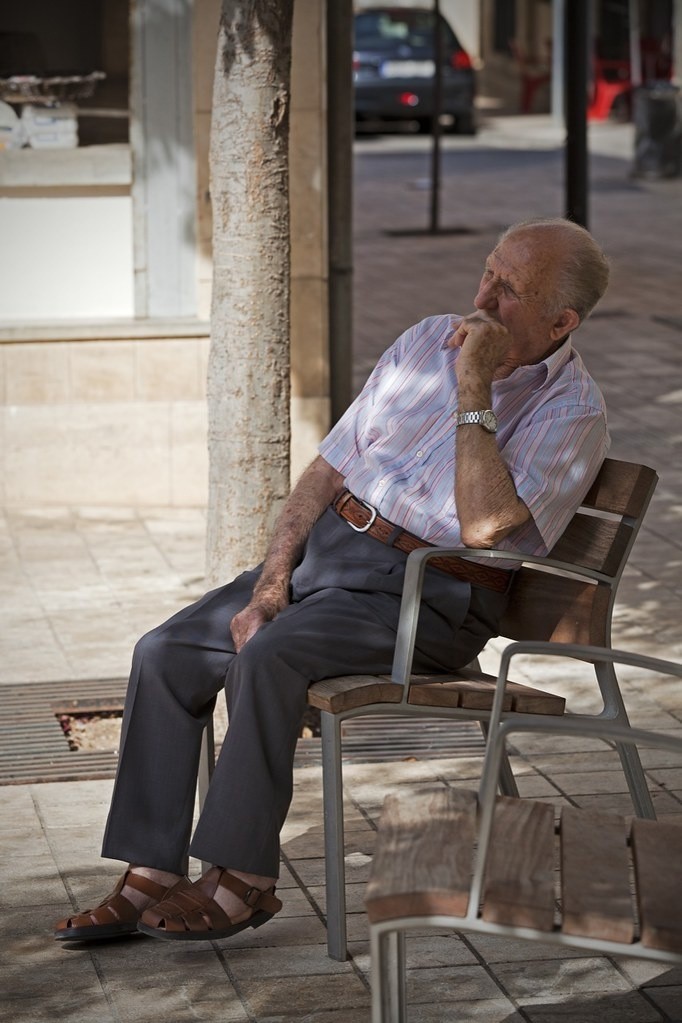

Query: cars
[349,5,481,137]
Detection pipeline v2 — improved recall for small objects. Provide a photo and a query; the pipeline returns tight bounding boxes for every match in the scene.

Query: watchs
[456,409,499,433]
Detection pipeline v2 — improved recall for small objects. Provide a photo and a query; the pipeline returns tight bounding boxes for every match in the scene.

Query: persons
[52,217,612,942]
[587,54,629,120]
[633,52,682,178]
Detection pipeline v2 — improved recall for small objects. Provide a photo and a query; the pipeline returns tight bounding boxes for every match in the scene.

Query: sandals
[137,868,283,941]
[54,864,193,943]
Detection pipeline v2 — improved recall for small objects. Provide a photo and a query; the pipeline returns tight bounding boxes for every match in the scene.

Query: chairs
[198,459,660,963]
[360,642,682,1023]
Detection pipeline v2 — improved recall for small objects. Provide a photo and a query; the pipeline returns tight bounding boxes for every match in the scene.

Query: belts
[336,491,511,593]
